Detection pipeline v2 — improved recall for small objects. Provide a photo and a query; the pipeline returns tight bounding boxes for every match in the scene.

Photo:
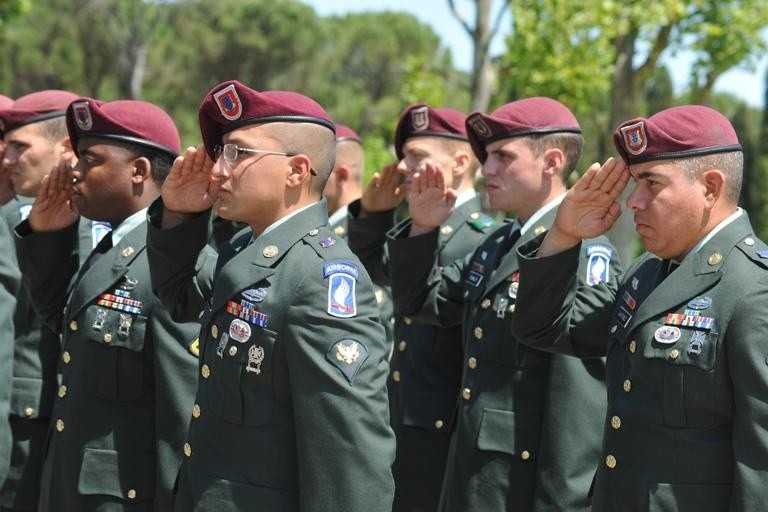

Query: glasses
[212,143,316,174]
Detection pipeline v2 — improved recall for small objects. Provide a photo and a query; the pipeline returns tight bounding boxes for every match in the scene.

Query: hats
[199,79,364,162]
[0,90,181,157]
[394,95,581,165]
[614,105,743,164]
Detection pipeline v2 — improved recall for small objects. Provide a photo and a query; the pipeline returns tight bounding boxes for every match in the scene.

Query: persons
[10,97,202,511]
[345,101,514,512]
[145,79,399,511]
[0,88,117,510]
[385,95,621,510]
[510,105,767,511]
[0,78,16,137]
[324,122,394,402]
